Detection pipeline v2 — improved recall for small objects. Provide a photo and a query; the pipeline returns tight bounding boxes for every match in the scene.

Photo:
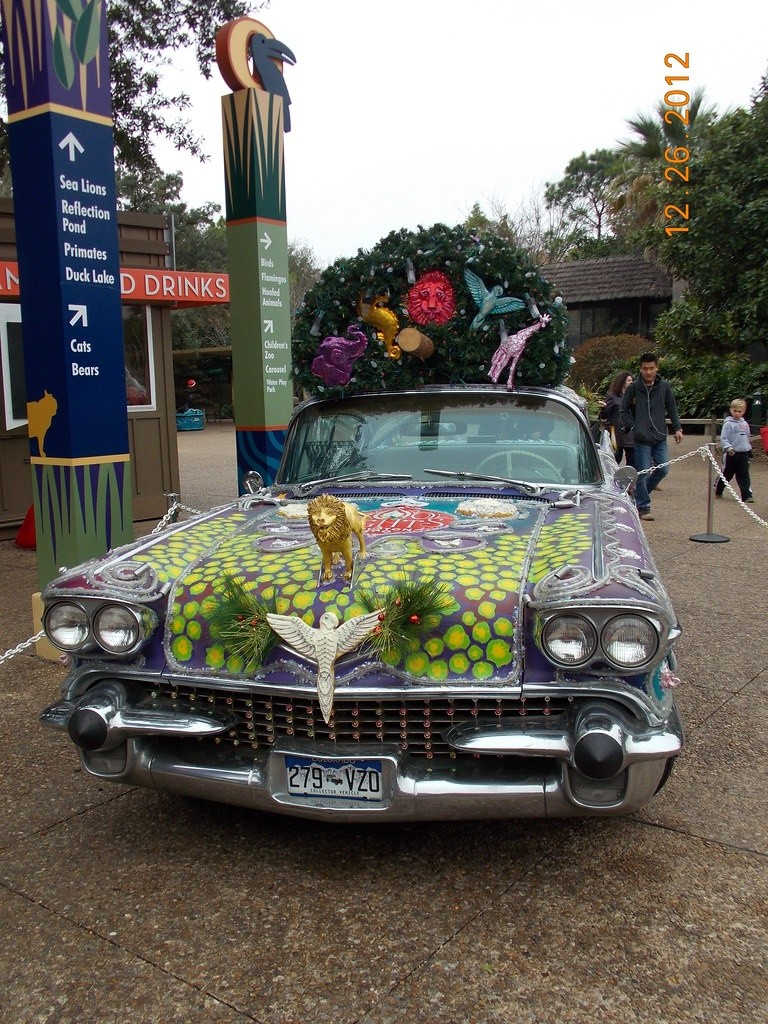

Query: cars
[38,374,686,828]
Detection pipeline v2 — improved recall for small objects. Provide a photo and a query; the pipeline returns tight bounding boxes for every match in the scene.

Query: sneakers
[638,507,654,522]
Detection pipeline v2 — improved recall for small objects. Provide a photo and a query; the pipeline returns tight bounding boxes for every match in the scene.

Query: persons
[597,372,636,496]
[713,399,756,503]
[620,352,684,521]
[476,418,554,443]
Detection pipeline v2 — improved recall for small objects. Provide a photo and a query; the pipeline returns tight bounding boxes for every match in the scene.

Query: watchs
[676,429,683,432]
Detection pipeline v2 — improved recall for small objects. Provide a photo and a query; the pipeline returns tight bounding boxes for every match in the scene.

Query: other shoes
[742,495,754,503]
[714,490,724,499]
